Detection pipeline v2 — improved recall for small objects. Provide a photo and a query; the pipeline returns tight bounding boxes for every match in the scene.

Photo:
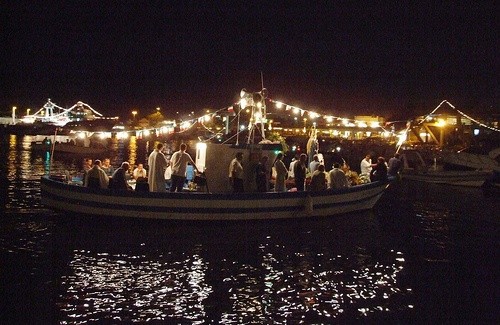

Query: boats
[39,86,393,221]
[395,163,492,187]
[425,145,500,173]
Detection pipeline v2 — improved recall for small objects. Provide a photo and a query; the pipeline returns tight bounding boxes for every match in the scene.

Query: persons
[359,152,377,176]
[294,153,308,191]
[256,155,270,192]
[309,155,321,178]
[133,161,146,180]
[170,143,203,192]
[101,158,114,176]
[273,153,290,191]
[41,135,100,149]
[369,156,388,182]
[86,159,110,190]
[387,152,401,176]
[226,152,246,192]
[164,164,173,179]
[327,163,348,189]
[308,164,327,191]
[148,142,171,193]
[84,158,93,174]
[289,154,301,179]
[111,161,134,191]
